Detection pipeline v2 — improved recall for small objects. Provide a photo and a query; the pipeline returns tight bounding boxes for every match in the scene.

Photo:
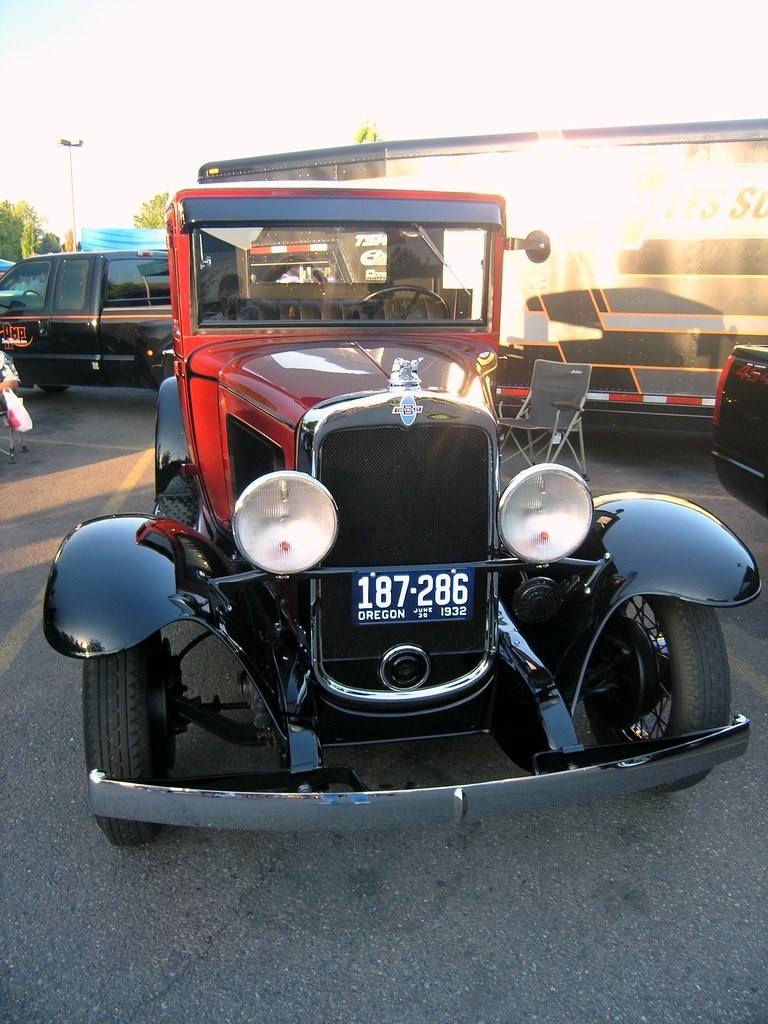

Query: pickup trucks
[0,250,175,393]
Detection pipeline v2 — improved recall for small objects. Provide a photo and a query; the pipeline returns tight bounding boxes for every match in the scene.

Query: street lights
[61,138,84,249]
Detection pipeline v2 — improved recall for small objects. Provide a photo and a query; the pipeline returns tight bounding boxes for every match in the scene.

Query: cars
[712,345,768,520]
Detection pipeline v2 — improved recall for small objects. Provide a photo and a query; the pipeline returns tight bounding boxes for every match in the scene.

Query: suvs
[41,180,761,849]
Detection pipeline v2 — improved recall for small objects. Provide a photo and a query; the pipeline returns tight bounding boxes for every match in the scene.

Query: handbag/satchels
[2,387,33,433]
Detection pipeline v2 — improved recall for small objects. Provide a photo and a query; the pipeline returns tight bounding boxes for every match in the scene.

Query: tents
[0,259,16,271]
[81,227,166,252]
[137,259,169,306]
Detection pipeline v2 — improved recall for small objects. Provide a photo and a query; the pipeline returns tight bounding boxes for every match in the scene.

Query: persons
[276,268,334,282]
[0,350,21,414]
[209,274,277,321]
[0,272,47,295]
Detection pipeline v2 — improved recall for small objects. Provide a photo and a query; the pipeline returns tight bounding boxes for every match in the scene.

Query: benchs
[228,296,451,321]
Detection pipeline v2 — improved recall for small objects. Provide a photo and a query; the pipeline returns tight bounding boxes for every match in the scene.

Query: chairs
[0,390,29,464]
[496,358,593,482]
